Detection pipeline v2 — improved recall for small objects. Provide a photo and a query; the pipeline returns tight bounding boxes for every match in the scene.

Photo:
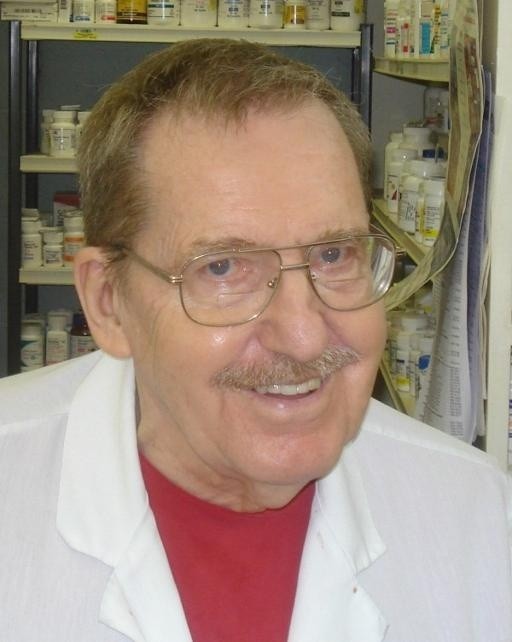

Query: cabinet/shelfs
[371,2,489,414]
[6,3,368,373]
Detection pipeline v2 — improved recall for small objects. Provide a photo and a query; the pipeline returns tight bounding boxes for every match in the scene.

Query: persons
[0,37,510,641]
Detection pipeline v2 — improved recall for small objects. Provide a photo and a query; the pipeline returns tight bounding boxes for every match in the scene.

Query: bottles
[17,105,95,372]
[384,1,448,415]
[69,1,366,32]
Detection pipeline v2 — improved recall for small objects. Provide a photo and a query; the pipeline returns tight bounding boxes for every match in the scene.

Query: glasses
[119,222,407,329]
